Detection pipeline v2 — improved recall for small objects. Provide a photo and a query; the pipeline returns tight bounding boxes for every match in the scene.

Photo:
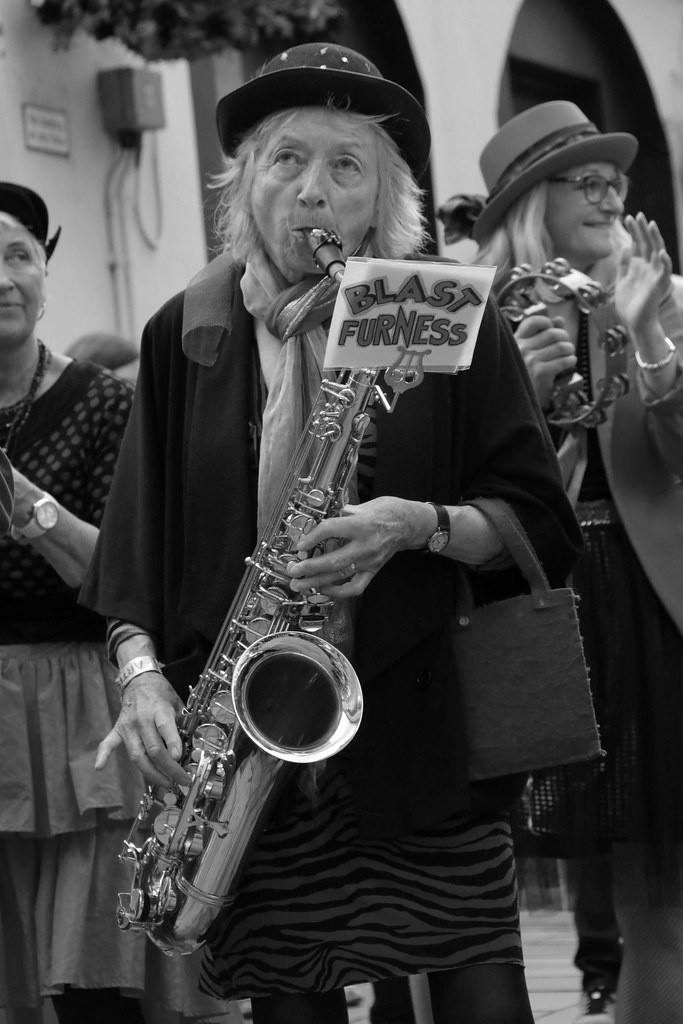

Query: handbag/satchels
[448,495,604,782]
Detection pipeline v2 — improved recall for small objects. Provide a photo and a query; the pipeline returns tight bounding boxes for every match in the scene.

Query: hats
[216,42,431,181]
[472,100,638,245]
[0,181,49,249]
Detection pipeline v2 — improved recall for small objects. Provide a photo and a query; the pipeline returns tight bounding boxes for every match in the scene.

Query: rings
[350,563,356,574]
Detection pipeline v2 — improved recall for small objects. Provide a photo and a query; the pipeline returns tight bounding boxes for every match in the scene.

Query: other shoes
[579,957,618,994]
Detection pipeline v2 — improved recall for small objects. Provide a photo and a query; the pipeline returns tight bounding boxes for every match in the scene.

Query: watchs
[408,501,451,554]
[11,491,59,540]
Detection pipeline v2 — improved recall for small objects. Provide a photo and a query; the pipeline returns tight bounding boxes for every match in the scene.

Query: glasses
[543,172,630,206]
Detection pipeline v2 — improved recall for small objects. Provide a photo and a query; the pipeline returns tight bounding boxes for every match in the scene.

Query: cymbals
[504,258,631,434]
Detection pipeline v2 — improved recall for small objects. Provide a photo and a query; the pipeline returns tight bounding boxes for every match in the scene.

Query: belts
[575,500,619,526]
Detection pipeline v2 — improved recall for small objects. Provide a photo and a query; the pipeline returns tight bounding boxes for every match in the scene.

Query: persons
[92,43,592,1024]
[0,182,245,1024]
[437,99,682,1024]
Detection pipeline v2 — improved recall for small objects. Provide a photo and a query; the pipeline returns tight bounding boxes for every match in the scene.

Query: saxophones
[114,223,429,956]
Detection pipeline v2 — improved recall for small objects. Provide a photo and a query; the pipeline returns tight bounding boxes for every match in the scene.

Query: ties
[569,304,604,488]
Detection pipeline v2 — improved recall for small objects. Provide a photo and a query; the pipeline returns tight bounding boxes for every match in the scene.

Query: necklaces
[0,338,51,465]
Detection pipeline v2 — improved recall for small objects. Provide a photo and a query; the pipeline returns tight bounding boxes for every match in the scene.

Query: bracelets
[634,337,676,370]
[115,655,162,702]
